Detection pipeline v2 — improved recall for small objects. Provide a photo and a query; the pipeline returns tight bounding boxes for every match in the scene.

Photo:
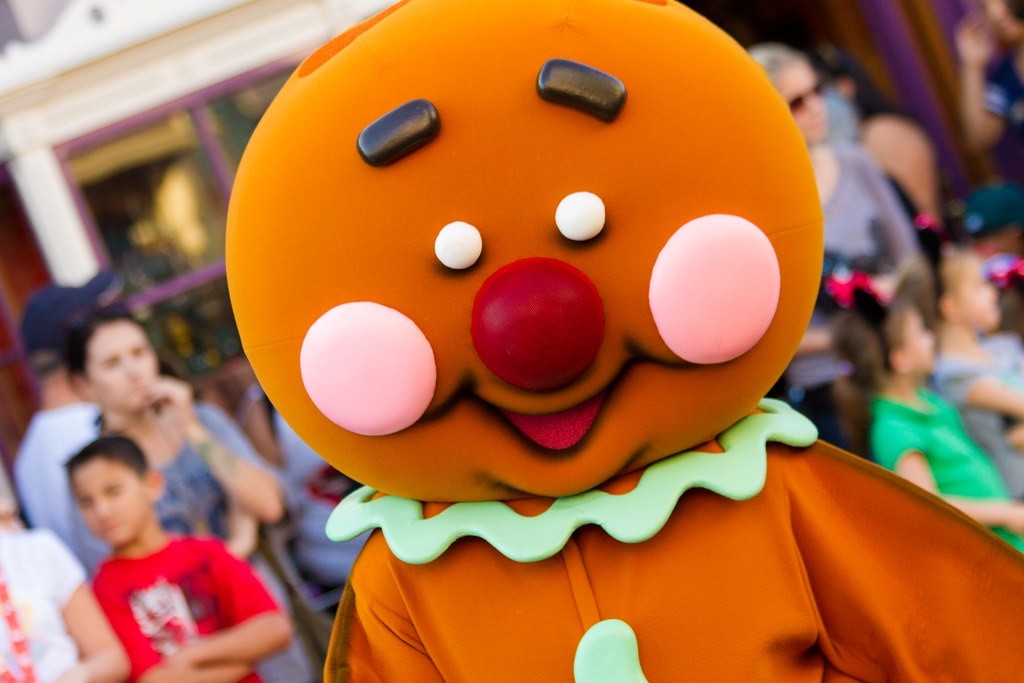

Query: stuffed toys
[223,0,1024,683]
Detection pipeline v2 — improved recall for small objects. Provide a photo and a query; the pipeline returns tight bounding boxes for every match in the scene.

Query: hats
[22,268,115,352]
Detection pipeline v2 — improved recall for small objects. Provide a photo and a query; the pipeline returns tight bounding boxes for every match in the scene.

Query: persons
[0,268,378,683]
[745,0,1024,557]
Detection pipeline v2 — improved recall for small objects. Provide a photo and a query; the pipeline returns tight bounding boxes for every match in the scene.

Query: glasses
[786,83,823,112]
